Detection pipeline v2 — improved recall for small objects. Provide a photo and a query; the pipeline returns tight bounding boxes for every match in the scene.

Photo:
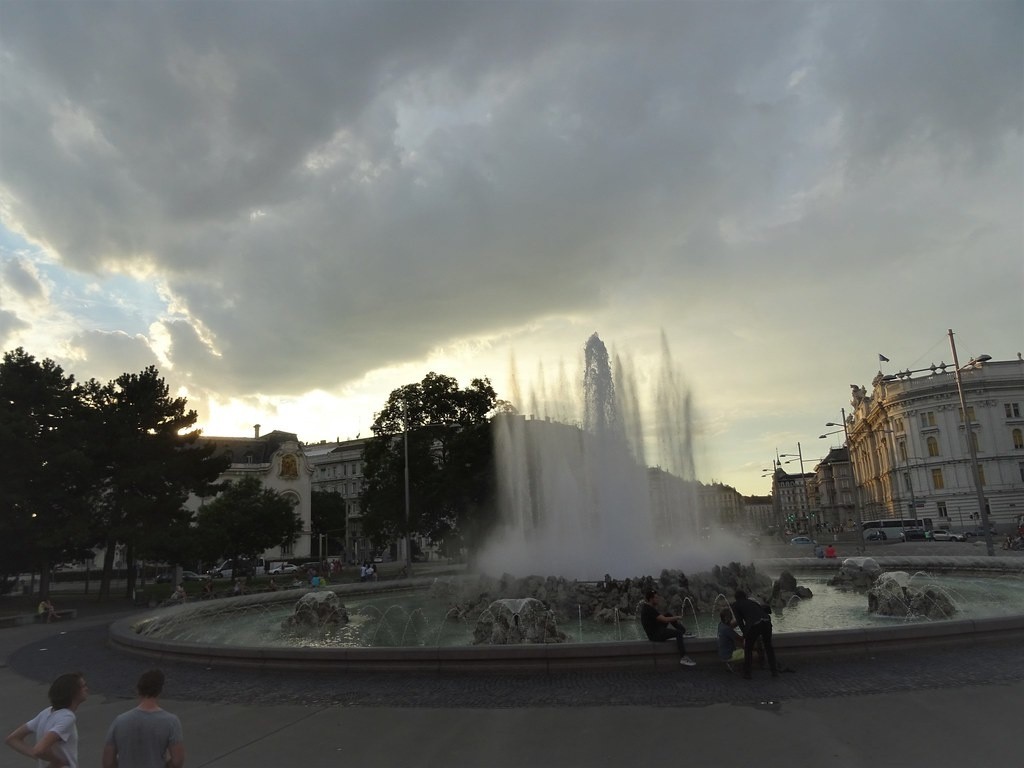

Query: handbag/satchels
[170,592,178,600]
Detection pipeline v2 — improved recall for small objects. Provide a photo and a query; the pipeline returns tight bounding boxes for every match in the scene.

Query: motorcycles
[1002,532,1024,551]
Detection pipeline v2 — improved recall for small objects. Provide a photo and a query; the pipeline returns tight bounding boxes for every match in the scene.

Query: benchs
[353,576,385,583]
[41,609,77,624]
[145,584,306,608]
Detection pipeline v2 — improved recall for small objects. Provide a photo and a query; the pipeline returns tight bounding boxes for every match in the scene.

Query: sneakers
[680,656,696,665]
[682,632,695,638]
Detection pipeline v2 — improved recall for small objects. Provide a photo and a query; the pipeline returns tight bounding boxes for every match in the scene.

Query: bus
[860,517,934,540]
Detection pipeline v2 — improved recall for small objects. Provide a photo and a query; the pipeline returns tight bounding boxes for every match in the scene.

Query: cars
[790,537,816,544]
[928,529,966,542]
[155,570,212,584]
[373,556,383,563]
[964,525,997,539]
[268,563,301,575]
[867,530,887,541]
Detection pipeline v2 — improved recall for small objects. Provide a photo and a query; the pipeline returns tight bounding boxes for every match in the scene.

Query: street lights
[819,407,893,532]
[761,459,786,539]
[880,329,996,557]
[780,442,835,544]
[370,396,461,577]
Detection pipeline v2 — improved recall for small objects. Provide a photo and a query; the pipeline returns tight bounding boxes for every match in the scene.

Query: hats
[645,590,656,601]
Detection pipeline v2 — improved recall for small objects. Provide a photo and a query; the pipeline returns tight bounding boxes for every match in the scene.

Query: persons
[201,580,216,600]
[360,562,377,583]
[924,529,933,541]
[102,669,185,768]
[717,595,782,679]
[311,575,326,588]
[233,576,251,596]
[640,588,699,666]
[900,530,906,542]
[171,583,187,604]
[318,557,344,573]
[814,544,837,559]
[5,673,86,768]
[269,570,300,590]
[819,521,843,534]
[38,597,61,624]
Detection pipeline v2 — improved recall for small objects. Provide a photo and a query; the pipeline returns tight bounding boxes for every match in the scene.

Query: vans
[215,557,266,578]
[899,529,926,540]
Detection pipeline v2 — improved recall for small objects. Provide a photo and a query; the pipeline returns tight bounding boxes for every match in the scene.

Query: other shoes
[726,661,735,673]
[772,671,780,676]
[55,615,61,618]
[744,672,752,679]
